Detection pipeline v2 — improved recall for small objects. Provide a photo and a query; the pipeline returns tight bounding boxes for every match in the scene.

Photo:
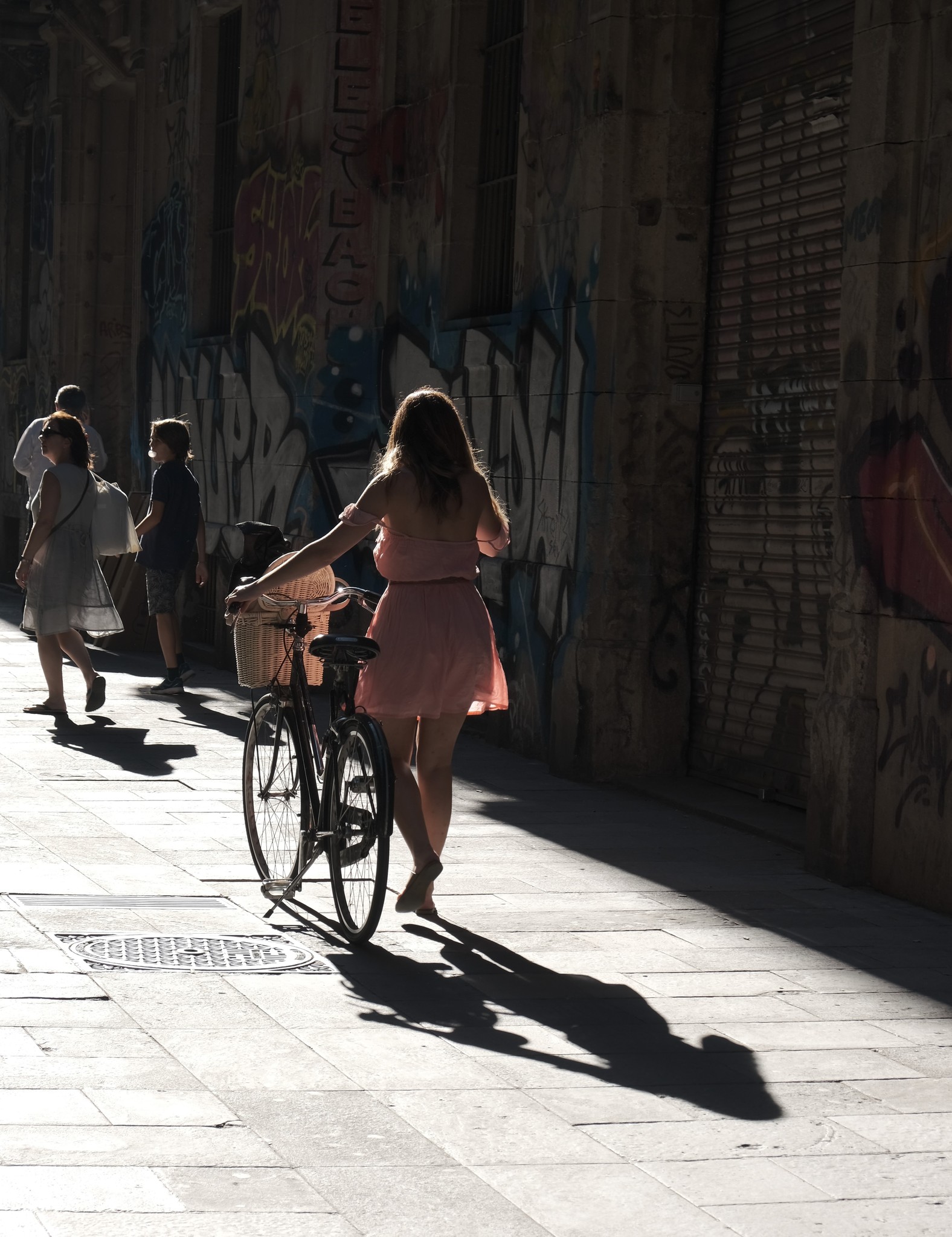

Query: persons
[116,418,209,691]
[8,416,140,714]
[12,386,106,635]
[221,386,511,916]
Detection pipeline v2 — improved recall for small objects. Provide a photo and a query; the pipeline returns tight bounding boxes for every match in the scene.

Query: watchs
[20,555,32,564]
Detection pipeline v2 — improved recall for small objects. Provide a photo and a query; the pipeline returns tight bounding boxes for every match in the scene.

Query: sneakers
[180,662,195,684]
[149,674,183,693]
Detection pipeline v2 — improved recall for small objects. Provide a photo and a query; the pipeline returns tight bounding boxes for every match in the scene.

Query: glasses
[40,427,68,440]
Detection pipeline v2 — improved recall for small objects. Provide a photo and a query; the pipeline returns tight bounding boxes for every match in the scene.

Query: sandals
[418,902,440,918]
[394,856,443,913]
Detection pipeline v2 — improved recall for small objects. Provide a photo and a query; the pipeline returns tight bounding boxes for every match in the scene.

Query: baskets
[234,545,336,689]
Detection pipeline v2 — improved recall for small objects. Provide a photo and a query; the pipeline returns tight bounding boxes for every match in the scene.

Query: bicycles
[222,572,399,949]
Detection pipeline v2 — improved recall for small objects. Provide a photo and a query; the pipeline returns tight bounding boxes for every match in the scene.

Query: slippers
[22,703,67,714]
[85,674,106,712]
